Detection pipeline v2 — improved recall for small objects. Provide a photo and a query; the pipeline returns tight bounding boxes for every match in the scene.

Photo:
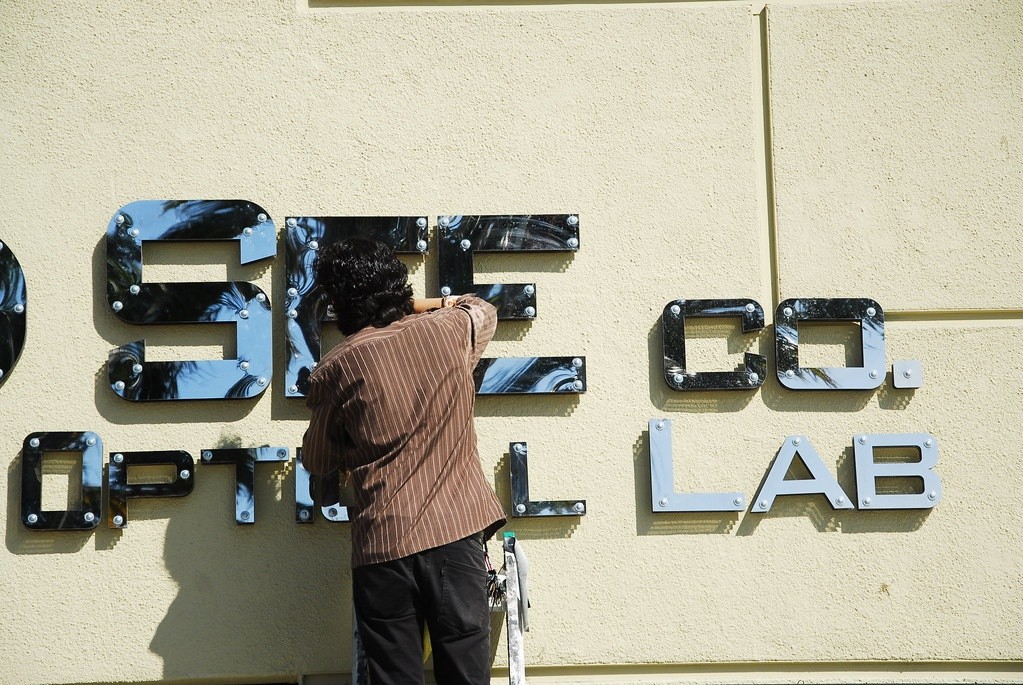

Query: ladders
[351,531,526,685]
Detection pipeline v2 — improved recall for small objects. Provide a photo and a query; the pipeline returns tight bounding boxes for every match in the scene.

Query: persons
[302,236,508,685]
